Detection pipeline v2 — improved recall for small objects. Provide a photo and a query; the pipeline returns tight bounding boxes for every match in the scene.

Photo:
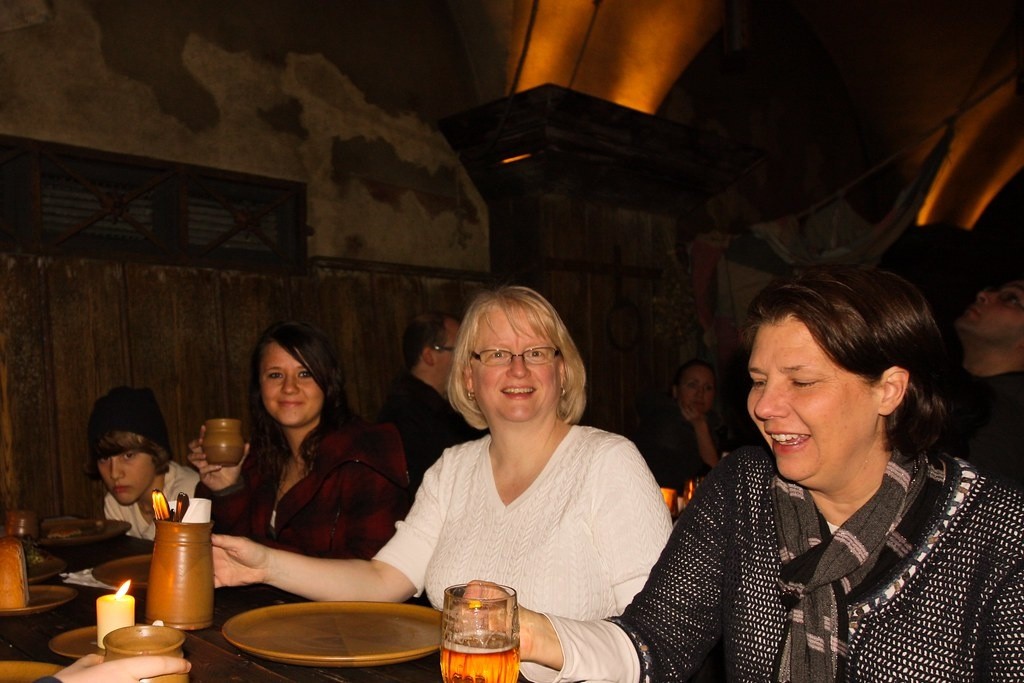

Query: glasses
[470,346,561,367]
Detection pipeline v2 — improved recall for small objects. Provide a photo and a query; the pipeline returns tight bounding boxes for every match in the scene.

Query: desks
[0,515,537,683]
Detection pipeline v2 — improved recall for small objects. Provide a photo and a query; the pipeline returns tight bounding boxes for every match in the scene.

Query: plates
[47,624,105,658]
[0,585,78,617]
[35,518,132,545]
[92,553,151,591]
[221,599,445,668]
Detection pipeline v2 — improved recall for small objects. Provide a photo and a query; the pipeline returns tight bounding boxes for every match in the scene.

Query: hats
[87,384,175,461]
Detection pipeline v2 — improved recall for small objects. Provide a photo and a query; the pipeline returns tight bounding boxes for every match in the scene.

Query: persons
[446,263,1024,683]
[954,280,1024,485]
[382,314,460,486]
[635,357,728,511]
[87,386,200,541]
[210,287,676,621]
[188,319,408,560]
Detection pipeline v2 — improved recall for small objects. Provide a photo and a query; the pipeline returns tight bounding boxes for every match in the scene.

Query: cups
[439,583,521,683]
[683,477,705,505]
[102,625,190,683]
[145,517,214,630]
[4,510,39,540]
[661,488,679,516]
[201,418,245,467]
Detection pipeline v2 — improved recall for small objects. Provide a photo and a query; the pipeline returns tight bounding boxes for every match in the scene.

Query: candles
[96,579,135,650]
